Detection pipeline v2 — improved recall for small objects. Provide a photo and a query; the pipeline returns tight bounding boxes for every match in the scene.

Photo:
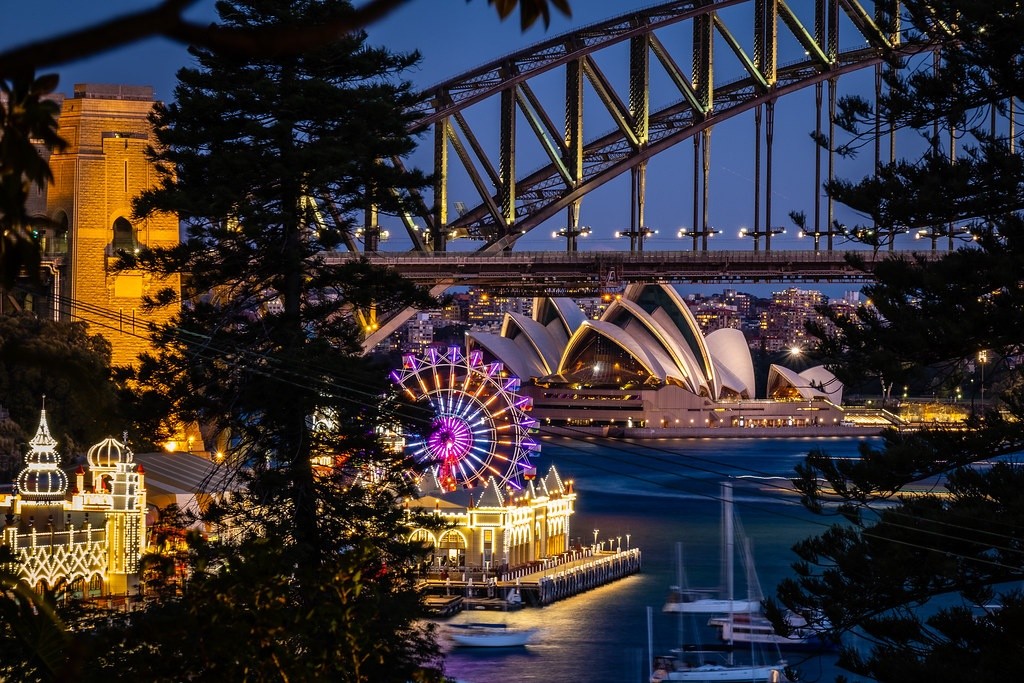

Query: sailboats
[645,482,838,683]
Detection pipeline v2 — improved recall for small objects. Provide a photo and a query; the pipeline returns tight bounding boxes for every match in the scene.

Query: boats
[447,626,539,647]
